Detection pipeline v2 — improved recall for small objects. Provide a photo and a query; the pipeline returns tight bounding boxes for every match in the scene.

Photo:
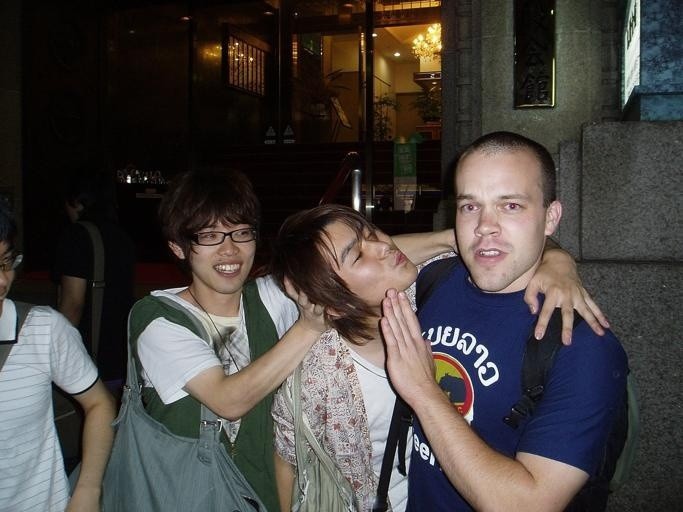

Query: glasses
[189,228,258,246]
[0,251,24,271]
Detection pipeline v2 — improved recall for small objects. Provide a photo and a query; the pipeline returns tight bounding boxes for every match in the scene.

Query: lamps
[408,22,443,57]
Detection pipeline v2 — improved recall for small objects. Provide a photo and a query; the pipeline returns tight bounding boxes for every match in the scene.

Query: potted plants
[408,93,444,128]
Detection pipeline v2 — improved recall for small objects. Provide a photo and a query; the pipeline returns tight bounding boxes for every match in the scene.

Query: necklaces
[186,287,238,371]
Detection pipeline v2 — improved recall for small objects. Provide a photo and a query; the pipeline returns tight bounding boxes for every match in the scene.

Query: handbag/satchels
[69,387,271,511]
[292,441,356,511]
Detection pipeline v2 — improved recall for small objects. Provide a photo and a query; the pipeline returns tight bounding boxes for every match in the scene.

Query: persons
[128,165,458,512]
[1,195,116,512]
[51,173,135,401]
[380,131,629,512]
[272,203,610,512]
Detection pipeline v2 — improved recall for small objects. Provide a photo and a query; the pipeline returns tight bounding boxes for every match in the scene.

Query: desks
[417,125,444,141]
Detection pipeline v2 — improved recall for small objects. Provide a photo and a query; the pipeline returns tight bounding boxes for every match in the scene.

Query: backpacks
[398,258,639,511]
